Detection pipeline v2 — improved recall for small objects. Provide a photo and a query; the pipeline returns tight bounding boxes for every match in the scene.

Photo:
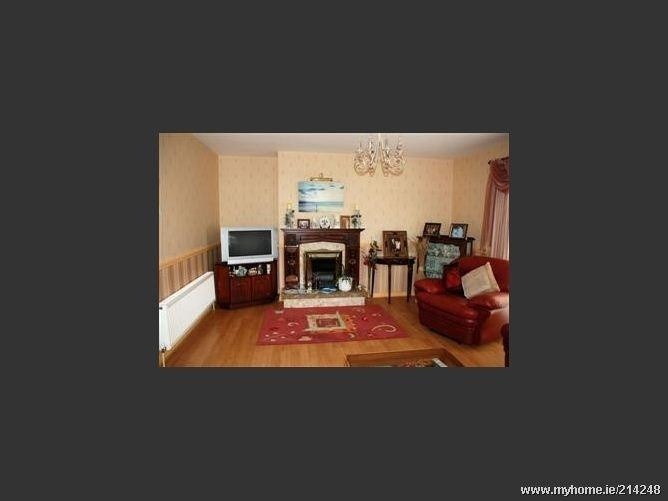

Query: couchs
[413,256,509,346]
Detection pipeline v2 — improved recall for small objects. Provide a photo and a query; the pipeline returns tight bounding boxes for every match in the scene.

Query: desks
[345,348,465,367]
[370,256,415,303]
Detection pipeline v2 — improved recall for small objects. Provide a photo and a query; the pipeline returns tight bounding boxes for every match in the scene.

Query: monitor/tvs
[220,226,279,266]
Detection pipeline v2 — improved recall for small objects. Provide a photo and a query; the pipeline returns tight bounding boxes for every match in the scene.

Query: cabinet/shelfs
[214,261,277,310]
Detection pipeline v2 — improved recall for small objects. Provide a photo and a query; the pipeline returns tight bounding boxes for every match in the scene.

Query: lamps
[354,133,408,177]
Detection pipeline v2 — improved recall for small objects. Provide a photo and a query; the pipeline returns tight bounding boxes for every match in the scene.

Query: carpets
[255,303,410,346]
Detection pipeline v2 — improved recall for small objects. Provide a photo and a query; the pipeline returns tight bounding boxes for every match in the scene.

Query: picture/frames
[340,215,350,229]
[449,223,468,239]
[297,219,310,229]
[383,231,409,258]
[423,223,441,237]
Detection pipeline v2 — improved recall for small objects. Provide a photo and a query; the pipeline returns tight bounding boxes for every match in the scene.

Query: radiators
[159,271,217,368]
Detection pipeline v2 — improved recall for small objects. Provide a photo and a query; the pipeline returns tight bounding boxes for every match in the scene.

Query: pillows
[443,265,461,291]
[461,262,500,300]
